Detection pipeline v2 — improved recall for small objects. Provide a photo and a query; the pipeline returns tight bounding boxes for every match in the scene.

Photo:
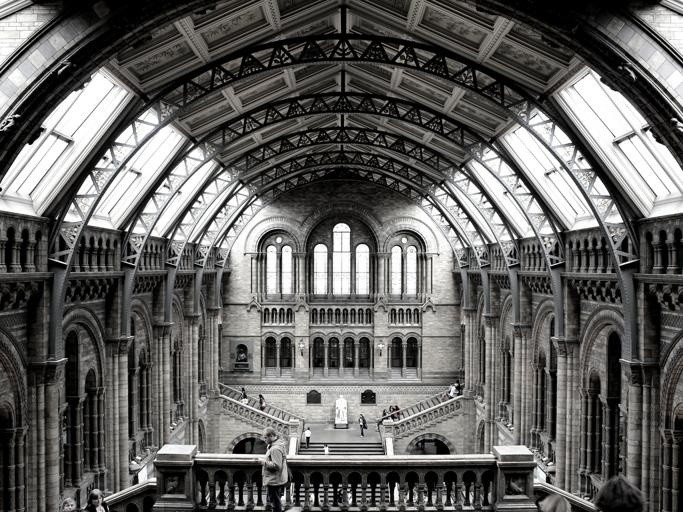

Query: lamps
[298,339,305,352]
[377,340,385,353]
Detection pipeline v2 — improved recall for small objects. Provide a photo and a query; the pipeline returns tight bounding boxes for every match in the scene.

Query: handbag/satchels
[242,399,249,403]
[287,468,294,482]
[363,424,367,429]
[262,402,267,408]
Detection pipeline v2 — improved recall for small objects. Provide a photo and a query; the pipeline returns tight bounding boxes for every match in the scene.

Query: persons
[595,475,646,511]
[359,414,366,438]
[85,488,111,512]
[241,387,248,405]
[448,379,461,399]
[382,405,400,422]
[259,394,266,411]
[62,497,76,512]
[323,444,329,454]
[304,427,311,449]
[257,427,289,511]
